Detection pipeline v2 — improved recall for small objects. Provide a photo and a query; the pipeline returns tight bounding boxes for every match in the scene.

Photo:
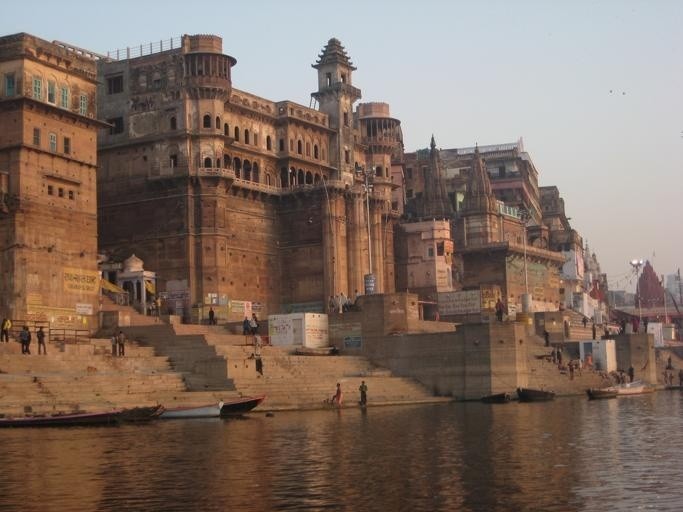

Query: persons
[662,356,683,385]
[250,312,259,335]
[328,289,360,312]
[20,326,30,354]
[552,350,634,385]
[0,317,11,341]
[208,306,215,326]
[109,334,117,354]
[118,331,125,355]
[582,315,640,339]
[24,327,30,352]
[252,332,263,355]
[359,380,367,407]
[496,297,506,321]
[243,316,249,335]
[543,330,551,346]
[36,326,47,355]
[332,382,343,408]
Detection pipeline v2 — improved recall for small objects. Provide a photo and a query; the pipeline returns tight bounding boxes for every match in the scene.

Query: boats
[584,388,617,400]
[0,395,264,426]
[601,380,657,394]
[481,392,511,403]
[516,387,555,403]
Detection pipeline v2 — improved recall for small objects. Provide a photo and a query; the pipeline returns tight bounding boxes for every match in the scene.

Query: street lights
[356,168,377,273]
[516,208,532,293]
[629,259,644,319]
